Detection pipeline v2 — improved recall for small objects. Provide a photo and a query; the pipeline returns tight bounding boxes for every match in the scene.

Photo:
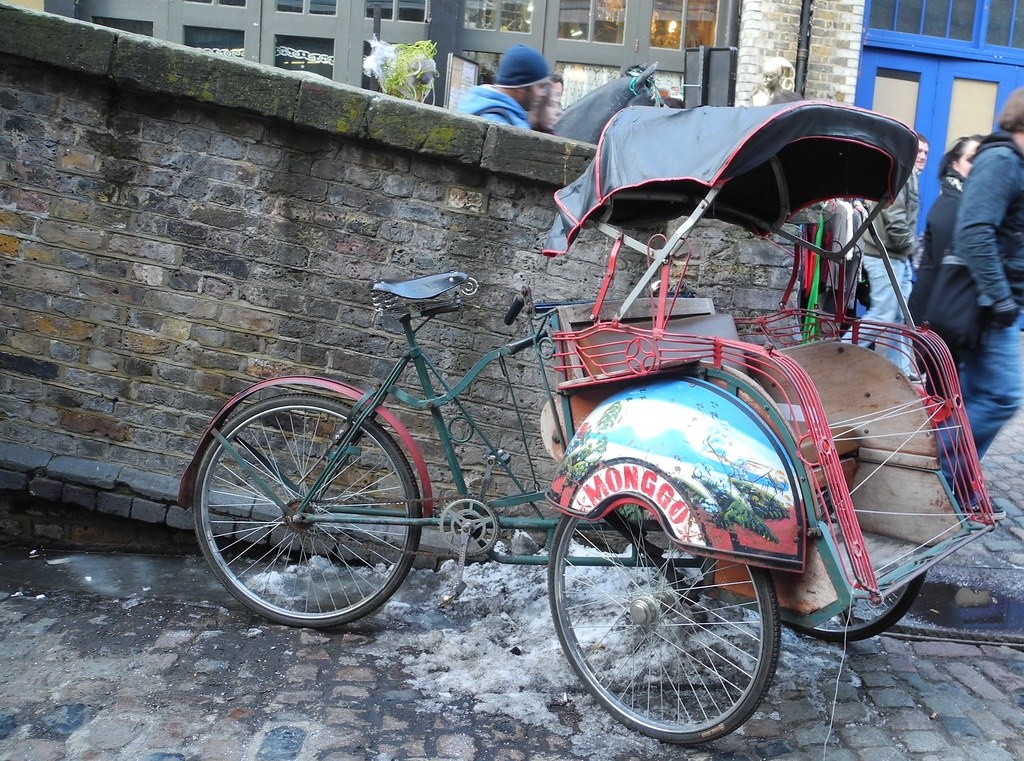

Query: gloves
[988,295,1019,329]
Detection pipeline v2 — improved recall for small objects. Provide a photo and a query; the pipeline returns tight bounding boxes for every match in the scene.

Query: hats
[495,43,551,88]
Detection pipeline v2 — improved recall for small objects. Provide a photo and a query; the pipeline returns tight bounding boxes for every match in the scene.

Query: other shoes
[907,372,926,384]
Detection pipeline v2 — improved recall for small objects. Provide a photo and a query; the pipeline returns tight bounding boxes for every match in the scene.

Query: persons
[456,44,564,134]
[840,89,1024,522]
[749,56,795,106]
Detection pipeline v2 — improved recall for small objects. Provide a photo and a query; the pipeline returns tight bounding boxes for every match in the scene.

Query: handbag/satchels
[923,254,1007,349]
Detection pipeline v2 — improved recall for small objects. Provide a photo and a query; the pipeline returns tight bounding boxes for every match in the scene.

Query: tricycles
[179,99,1003,745]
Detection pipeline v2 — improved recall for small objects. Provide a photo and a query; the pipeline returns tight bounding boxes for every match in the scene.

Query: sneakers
[960,497,1006,520]
